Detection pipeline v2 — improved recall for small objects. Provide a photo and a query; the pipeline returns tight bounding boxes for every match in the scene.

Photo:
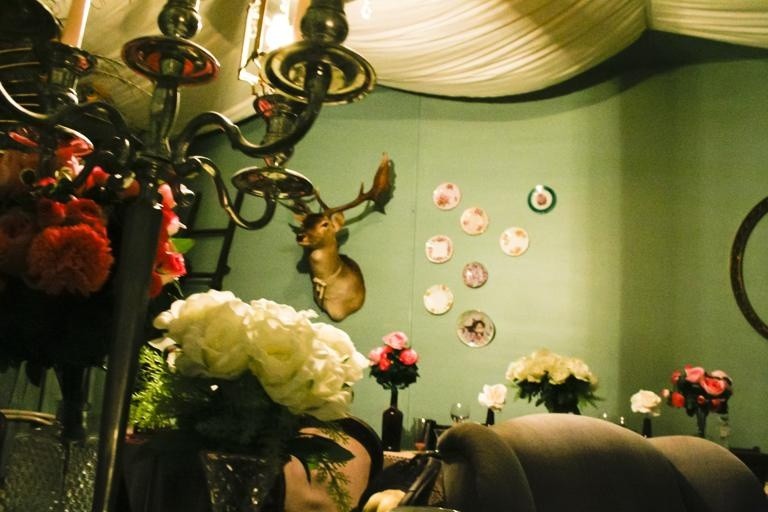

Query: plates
[422,181,556,349]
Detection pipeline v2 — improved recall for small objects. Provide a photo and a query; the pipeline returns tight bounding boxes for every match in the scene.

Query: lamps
[0,0,377,512]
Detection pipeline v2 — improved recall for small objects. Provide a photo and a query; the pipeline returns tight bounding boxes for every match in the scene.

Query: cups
[450,403,470,423]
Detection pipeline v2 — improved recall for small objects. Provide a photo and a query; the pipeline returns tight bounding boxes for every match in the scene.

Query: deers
[278,181,373,324]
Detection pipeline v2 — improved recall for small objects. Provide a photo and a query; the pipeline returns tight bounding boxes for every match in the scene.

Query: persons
[463,319,488,345]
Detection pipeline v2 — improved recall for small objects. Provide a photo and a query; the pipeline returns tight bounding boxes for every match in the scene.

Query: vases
[200,444,281,512]
[487,410,494,425]
[642,419,653,438]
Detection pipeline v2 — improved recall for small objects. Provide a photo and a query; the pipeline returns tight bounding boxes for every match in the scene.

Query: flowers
[366,330,423,392]
[627,387,663,419]
[129,283,370,512]
[505,347,607,415]
[476,384,507,410]
[664,365,735,430]
[15,142,194,310]
